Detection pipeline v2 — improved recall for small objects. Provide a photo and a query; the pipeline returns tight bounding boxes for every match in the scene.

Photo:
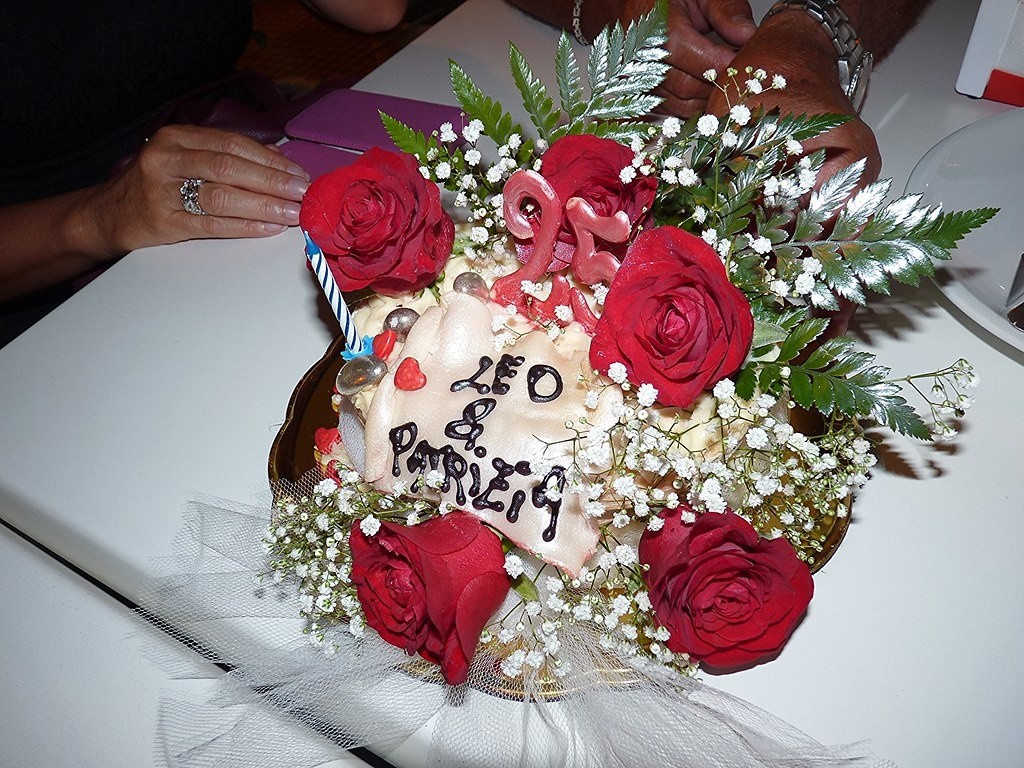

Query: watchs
[760,0,873,116]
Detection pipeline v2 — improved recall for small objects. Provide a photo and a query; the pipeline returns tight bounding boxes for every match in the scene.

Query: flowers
[265,0,1003,685]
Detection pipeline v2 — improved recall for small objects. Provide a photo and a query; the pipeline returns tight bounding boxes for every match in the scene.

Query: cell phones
[284,89,472,157]
[278,141,362,181]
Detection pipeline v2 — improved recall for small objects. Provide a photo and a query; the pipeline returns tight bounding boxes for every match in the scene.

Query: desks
[1,0,1024,768]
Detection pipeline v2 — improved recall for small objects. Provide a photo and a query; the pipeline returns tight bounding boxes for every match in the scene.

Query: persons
[0,0,408,302]
[502,0,936,241]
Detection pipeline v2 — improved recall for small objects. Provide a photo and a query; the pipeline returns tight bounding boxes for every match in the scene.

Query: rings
[180,178,208,215]
